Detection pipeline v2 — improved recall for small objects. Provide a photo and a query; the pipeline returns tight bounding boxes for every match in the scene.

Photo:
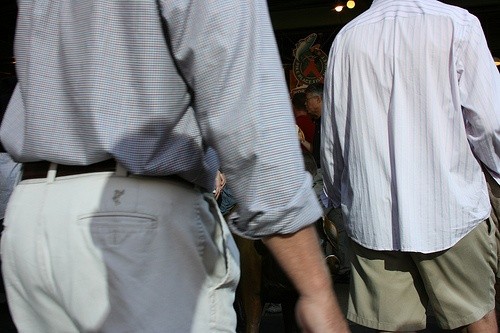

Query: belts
[20,158,205,192]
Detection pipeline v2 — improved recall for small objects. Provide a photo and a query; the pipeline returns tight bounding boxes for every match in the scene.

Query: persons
[0,0,350,333]
[320,0,500,333]
[292,80,352,283]
